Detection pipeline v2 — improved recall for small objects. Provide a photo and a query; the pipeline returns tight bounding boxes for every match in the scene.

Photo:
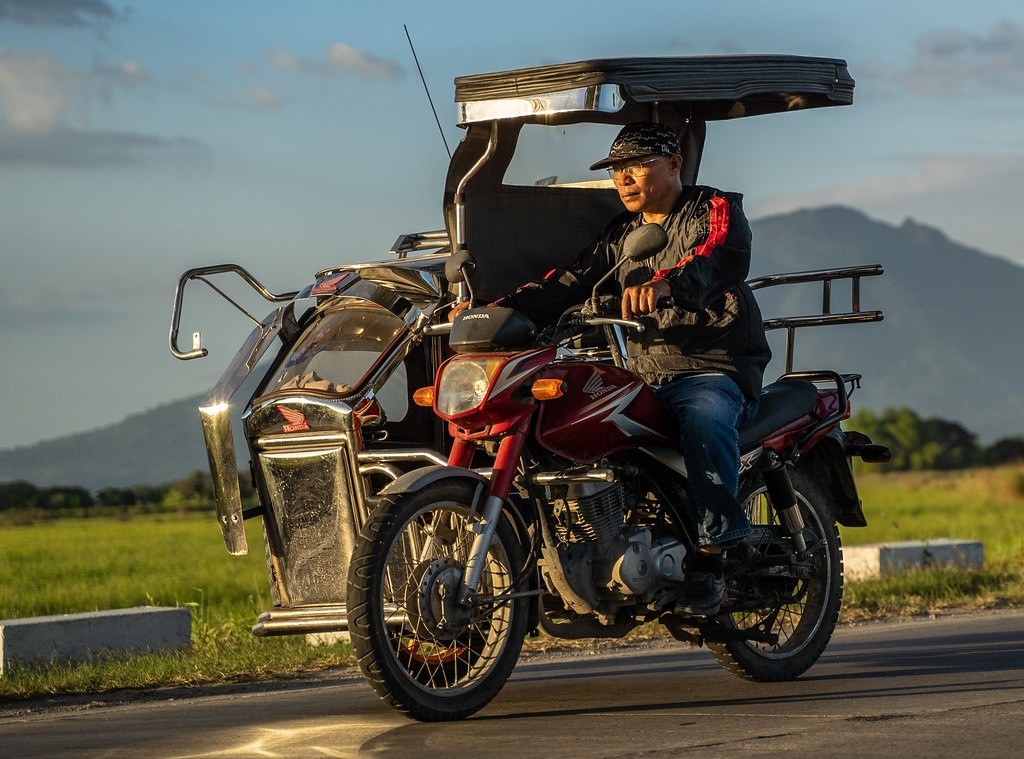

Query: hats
[588,119,686,171]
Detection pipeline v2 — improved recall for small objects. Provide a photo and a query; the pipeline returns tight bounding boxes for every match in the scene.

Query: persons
[448,122,772,618]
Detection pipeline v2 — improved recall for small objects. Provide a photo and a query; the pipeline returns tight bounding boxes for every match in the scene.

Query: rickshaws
[170,54,885,721]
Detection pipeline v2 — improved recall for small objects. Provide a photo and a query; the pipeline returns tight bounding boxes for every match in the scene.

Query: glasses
[605,155,671,179]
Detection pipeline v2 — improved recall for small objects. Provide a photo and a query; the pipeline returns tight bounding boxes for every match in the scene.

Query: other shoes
[671,570,728,616]
